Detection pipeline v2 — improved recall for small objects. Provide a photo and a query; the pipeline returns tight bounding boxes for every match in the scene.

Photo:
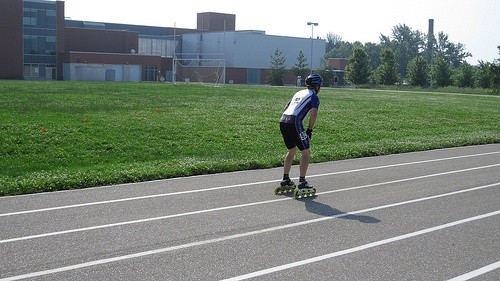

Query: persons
[279,72,323,189]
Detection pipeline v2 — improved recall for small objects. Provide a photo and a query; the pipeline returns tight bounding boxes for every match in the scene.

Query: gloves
[306,128,312,139]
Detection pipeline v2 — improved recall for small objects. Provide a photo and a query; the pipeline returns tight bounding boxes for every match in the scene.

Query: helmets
[305,74,324,86]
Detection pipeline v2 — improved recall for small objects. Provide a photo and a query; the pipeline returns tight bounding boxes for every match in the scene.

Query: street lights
[306,22,319,74]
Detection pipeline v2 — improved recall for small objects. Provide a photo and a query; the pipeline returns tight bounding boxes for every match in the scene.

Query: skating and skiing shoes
[274,178,296,194]
[294,180,316,198]
